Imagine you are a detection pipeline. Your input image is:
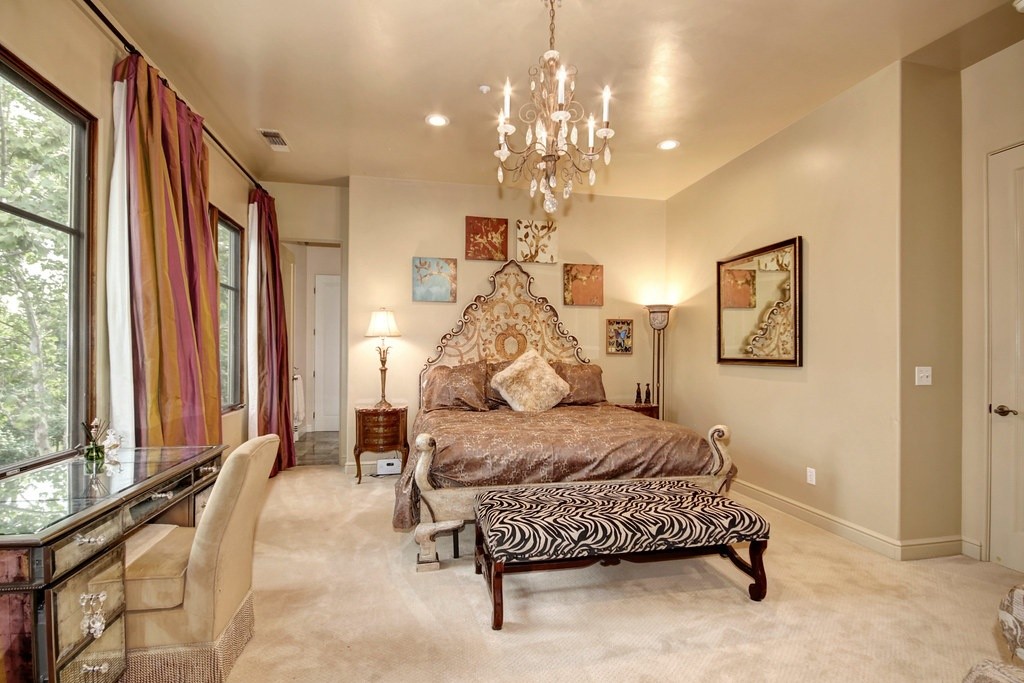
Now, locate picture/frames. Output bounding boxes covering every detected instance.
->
[604,317,635,357]
[712,234,805,370]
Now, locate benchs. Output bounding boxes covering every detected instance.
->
[465,478,782,633]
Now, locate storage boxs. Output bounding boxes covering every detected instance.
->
[376,457,404,477]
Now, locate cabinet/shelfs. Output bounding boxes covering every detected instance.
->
[0,443,233,683]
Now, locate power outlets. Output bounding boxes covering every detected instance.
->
[806,466,815,485]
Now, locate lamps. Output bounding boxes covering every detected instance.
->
[491,0,620,213]
[363,305,402,411]
[645,304,674,420]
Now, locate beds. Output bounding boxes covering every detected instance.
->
[391,259,741,590]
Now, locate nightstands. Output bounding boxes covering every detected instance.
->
[350,404,410,486]
[614,403,660,421]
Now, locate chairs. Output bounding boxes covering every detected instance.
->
[56,429,281,681]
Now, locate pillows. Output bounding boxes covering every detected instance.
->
[489,347,571,413]
[419,357,488,414]
[555,355,607,405]
[488,354,561,410]
